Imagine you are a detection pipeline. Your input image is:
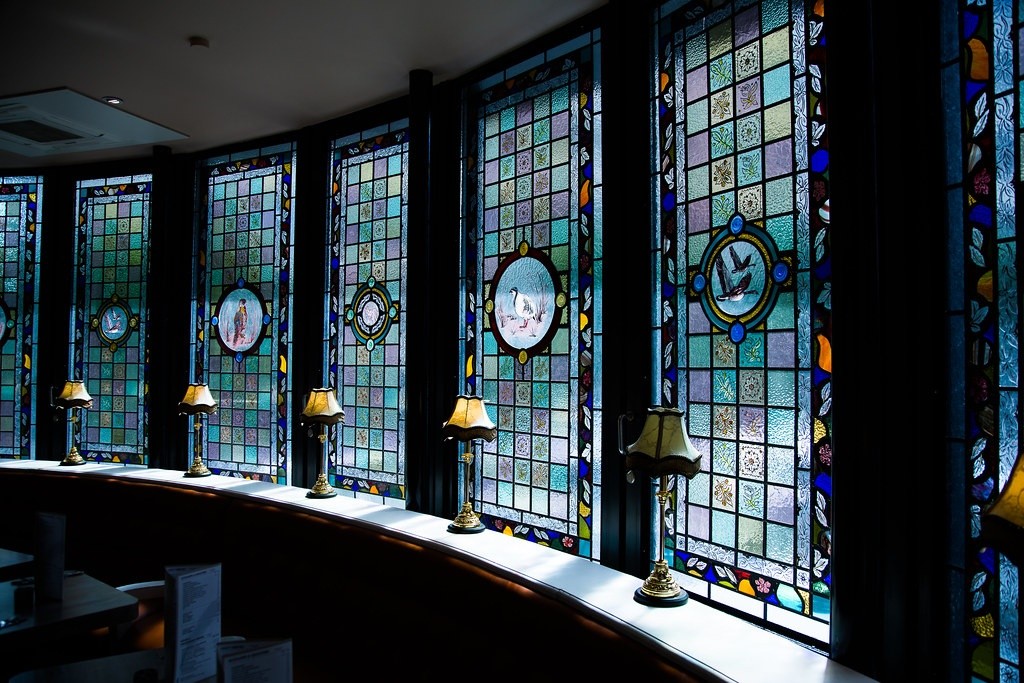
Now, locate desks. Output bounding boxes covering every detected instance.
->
[0,547,247,683]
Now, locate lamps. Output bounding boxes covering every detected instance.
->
[626,406,704,609]
[56,380,94,466]
[442,395,497,535]
[177,383,220,478]
[980,452,1024,683]
[299,388,346,499]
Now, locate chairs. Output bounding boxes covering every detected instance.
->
[92,579,166,654]
